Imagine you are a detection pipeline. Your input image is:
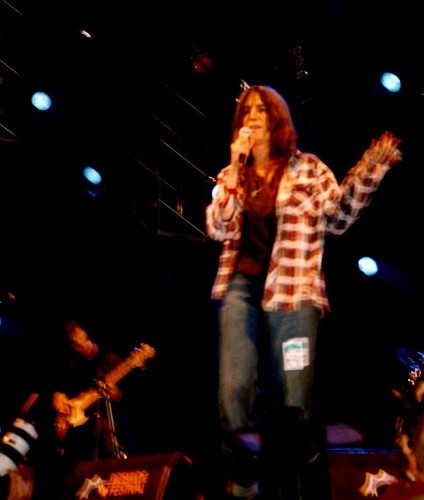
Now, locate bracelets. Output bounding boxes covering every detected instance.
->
[220,183,240,208]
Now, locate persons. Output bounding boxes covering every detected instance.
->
[206,83,400,432]
[52,326,126,459]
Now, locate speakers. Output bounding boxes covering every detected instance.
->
[73,453,194,500]
[330,448,409,500]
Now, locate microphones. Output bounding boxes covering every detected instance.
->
[238,127,251,167]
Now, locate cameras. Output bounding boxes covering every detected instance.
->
[1,394,55,480]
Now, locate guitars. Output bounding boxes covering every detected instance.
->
[54,341,155,440]
